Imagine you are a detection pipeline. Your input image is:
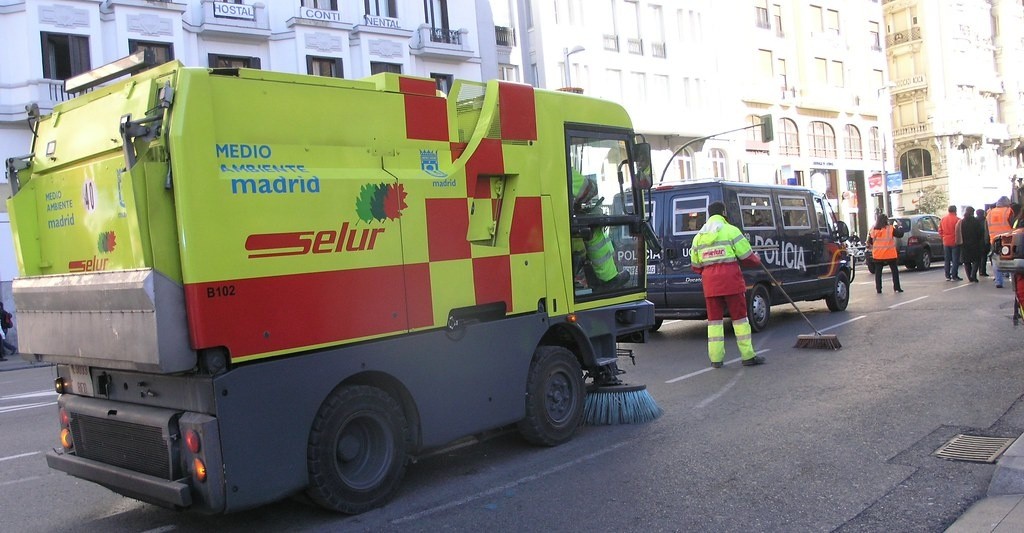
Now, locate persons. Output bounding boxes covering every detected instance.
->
[571,166,630,294]
[961,207,984,282]
[634,149,651,189]
[690,202,766,368]
[975,196,1021,288]
[850,232,861,247]
[0,301,17,361]
[868,214,904,293]
[938,205,963,281]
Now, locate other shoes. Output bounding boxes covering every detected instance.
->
[0,358,8,361]
[946,277,951,281]
[742,356,765,366]
[968,278,978,281]
[996,285,1002,288]
[980,273,989,276]
[606,269,630,289]
[877,289,881,293]
[951,277,963,282]
[894,288,903,292]
[11,347,16,354]
[710,361,723,368]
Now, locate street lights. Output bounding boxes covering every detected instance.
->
[564,46,585,86]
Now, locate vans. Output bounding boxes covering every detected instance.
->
[610,175,855,333]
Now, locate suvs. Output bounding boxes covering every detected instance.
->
[864,214,964,271]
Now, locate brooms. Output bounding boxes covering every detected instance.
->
[758,260,843,352]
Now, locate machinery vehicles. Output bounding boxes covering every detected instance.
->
[5,50,660,519]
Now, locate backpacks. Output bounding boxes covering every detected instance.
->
[4,312,14,328]
